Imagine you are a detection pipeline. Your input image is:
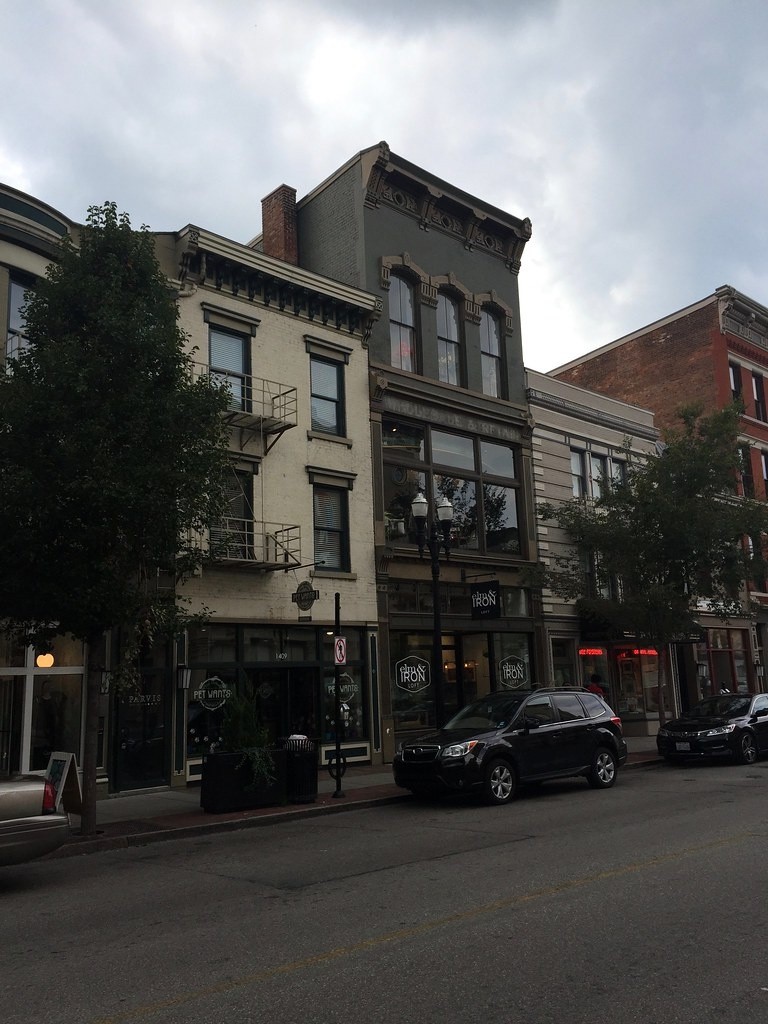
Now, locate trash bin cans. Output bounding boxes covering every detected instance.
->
[273,735,323,804]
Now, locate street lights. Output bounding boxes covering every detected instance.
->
[411,489,454,730]
[755,660,764,693]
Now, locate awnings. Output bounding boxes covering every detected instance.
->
[578,615,705,649]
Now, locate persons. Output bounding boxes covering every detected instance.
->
[588,674,604,701]
[719,682,730,695]
[120,684,146,752]
[38,681,59,755]
[460,667,477,707]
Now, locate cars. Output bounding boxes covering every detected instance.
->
[657,693,768,764]
[0,771,69,867]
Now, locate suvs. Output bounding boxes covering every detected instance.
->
[392,686,628,805]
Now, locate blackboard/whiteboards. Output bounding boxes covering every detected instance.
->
[45,752,71,807]
